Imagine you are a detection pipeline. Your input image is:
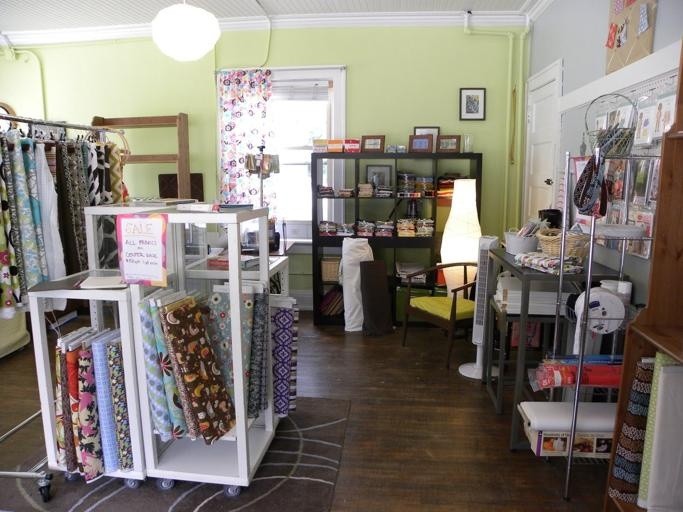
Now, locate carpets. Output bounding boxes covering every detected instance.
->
[0,397,351,512]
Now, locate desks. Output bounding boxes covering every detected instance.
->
[485,247,628,454]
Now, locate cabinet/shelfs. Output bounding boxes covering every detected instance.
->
[312,153,482,326]
[545,148,661,502]
[605,38,683,512]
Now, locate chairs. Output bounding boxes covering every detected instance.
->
[402,262,478,369]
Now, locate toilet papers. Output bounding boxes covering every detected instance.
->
[571,289,626,354]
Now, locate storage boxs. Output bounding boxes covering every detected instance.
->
[312,139,361,153]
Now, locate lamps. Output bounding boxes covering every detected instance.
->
[440,179,482,338]
[149,0,221,64]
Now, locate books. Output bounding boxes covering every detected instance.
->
[176,202,254,212]
[80,276,129,289]
[128,199,198,207]
[207,254,260,269]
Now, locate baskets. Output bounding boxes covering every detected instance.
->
[535,228,590,263]
[583,92,638,156]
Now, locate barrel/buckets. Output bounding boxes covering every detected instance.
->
[505,228,539,256]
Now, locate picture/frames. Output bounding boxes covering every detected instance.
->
[409,127,460,154]
[459,88,485,120]
[362,136,385,153]
[366,165,391,186]
